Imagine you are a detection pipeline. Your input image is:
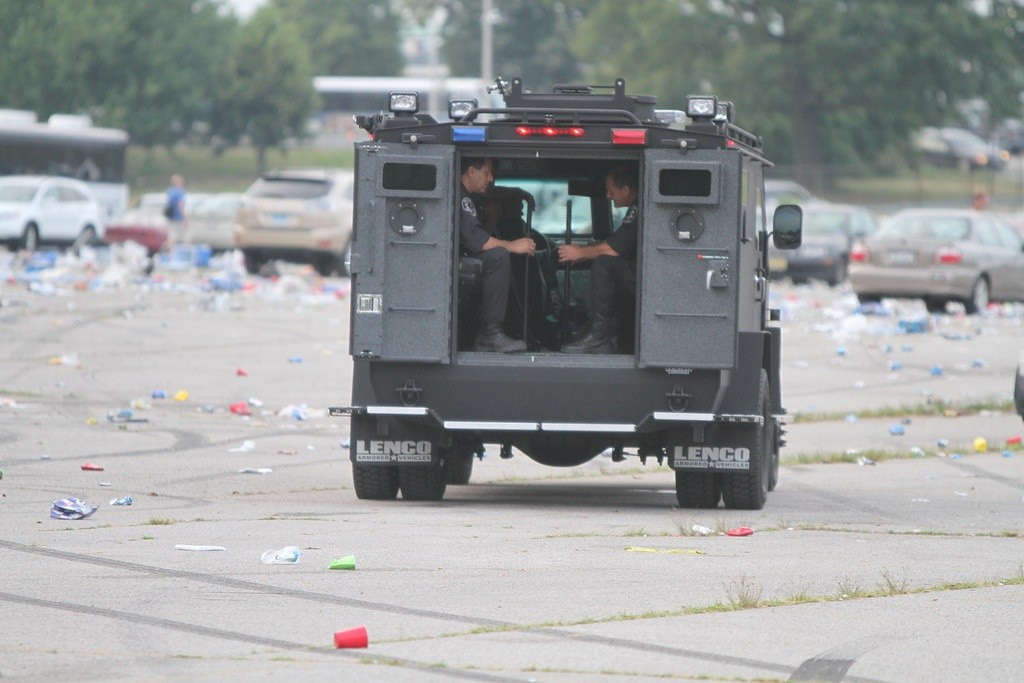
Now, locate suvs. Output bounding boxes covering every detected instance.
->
[234,168,354,276]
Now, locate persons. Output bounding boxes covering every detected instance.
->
[164,174,192,249]
[457,157,536,353]
[557,165,638,355]
[971,186,989,209]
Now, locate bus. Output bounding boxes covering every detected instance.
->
[0,110,131,249]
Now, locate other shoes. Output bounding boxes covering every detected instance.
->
[560,330,611,354]
[474,324,527,353]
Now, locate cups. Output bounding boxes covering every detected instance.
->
[329,555,355,570]
[973,437,987,452]
[334,627,367,650]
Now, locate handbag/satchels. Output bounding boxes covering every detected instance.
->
[164,205,174,218]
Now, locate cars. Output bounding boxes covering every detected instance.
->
[756,177,870,285]
[916,126,1013,169]
[851,207,1022,314]
[111,187,239,256]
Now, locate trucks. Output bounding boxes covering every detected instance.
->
[349,78,806,515]
